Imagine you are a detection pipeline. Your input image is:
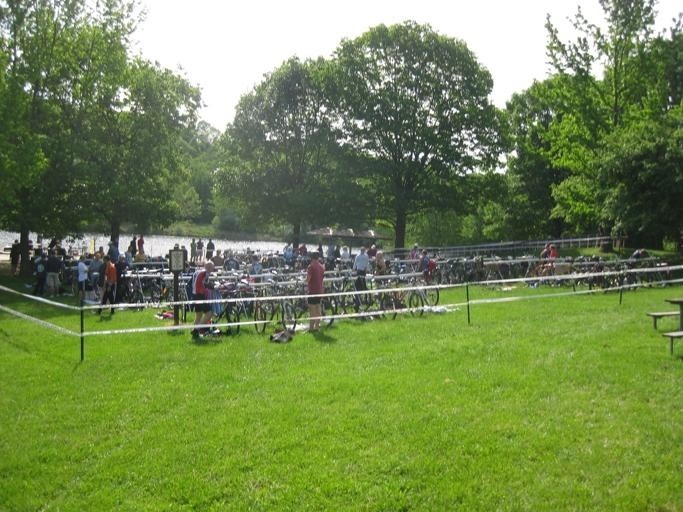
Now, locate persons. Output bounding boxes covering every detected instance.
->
[190,259,220,341]
[538,243,550,267]
[8,232,436,317]
[540,244,558,275]
[626,248,648,291]
[298,249,325,333]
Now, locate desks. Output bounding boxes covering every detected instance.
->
[664,299,683,331]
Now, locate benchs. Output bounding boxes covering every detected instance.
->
[662,331,683,356]
[646,311,680,330]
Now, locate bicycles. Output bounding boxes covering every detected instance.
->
[30,256,672,334]
